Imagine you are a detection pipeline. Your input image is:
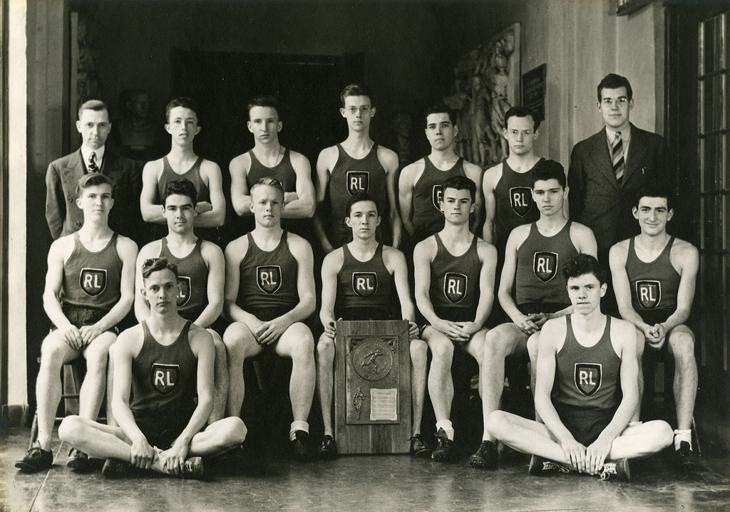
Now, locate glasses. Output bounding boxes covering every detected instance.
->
[602,97,628,108]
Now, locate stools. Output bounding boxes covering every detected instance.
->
[27,354,108,457]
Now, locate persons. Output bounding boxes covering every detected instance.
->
[608,184,698,478]
[316,84,403,254]
[399,103,483,251]
[134,177,229,467]
[486,254,674,479]
[223,177,316,463]
[568,73,674,319]
[45,100,141,416]
[413,176,506,469]
[57,258,247,478]
[483,106,566,325]
[105,90,168,181]
[14,174,138,472]
[316,193,433,460]
[140,97,226,253]
[455,39,511,169]
[469,160,597,471]
[229,95,317,255]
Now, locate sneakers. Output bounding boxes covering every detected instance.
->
[67,447,98,471]
[183,457,205,481]
[287,430,315,461]
[673,440,704,481]
[319,434,336,458]
[409,427,632,481]
[102,458,130,476]
[15,440,53,472]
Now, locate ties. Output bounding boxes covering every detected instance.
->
[610,130,627,186]
[87,152,100,174]
